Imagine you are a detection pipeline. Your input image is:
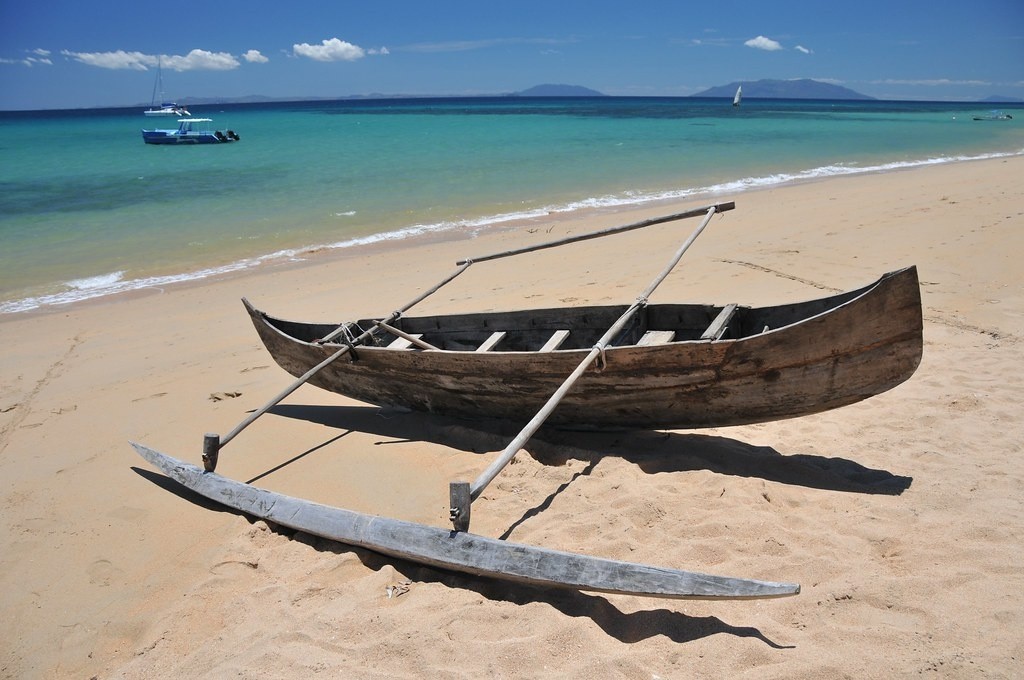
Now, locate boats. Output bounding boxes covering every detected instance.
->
[973,112,1013,121]
[141,118,240,145]
[240,263,922,435]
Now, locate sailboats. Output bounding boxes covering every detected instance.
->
[733,85,742,107]
[144,55,191,119]
